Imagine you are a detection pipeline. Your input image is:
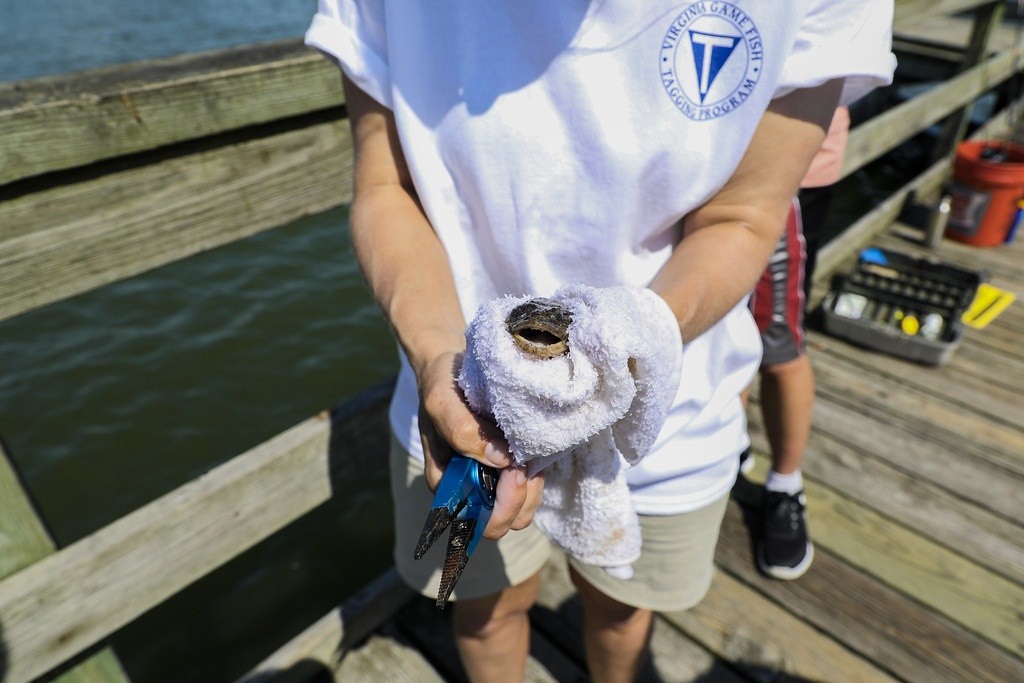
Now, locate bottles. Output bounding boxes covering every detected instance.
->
[925,196,951,248]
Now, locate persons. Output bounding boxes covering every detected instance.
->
[738,197,815,580]
[304,0,899,683]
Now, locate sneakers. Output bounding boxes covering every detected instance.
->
[739,447,754,474]
[756,486,813,580]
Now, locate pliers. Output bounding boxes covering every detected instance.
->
[413,451,498,609]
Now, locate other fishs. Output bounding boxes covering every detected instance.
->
[506,297,574,361]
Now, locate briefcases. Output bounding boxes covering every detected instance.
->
[820,244,981,367]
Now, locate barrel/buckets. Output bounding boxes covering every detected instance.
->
[944,141,1024,249]
